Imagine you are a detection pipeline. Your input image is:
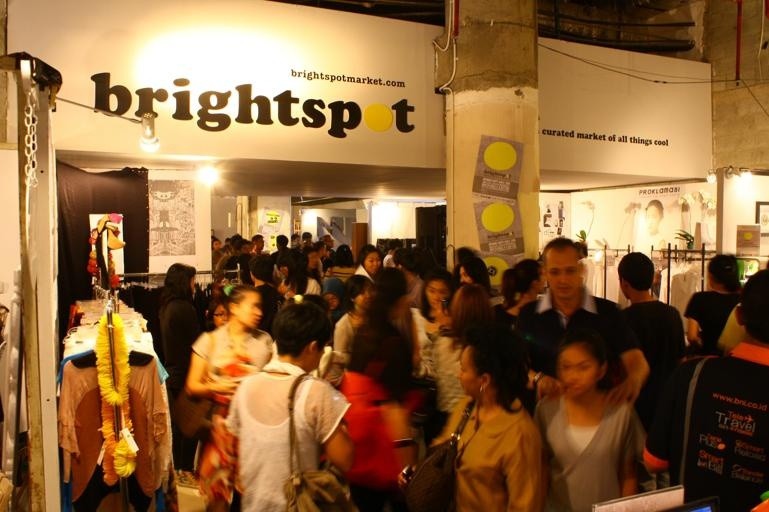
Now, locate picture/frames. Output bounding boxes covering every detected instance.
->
[756,201,769,236]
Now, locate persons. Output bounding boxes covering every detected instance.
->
[154,231,768,511]
[639,200,667,249]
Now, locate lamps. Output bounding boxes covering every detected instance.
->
[55,98,162,158]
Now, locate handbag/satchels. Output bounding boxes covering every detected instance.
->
[405,400,476,512]
[173,388,214,438]
[285,465,359,512]
